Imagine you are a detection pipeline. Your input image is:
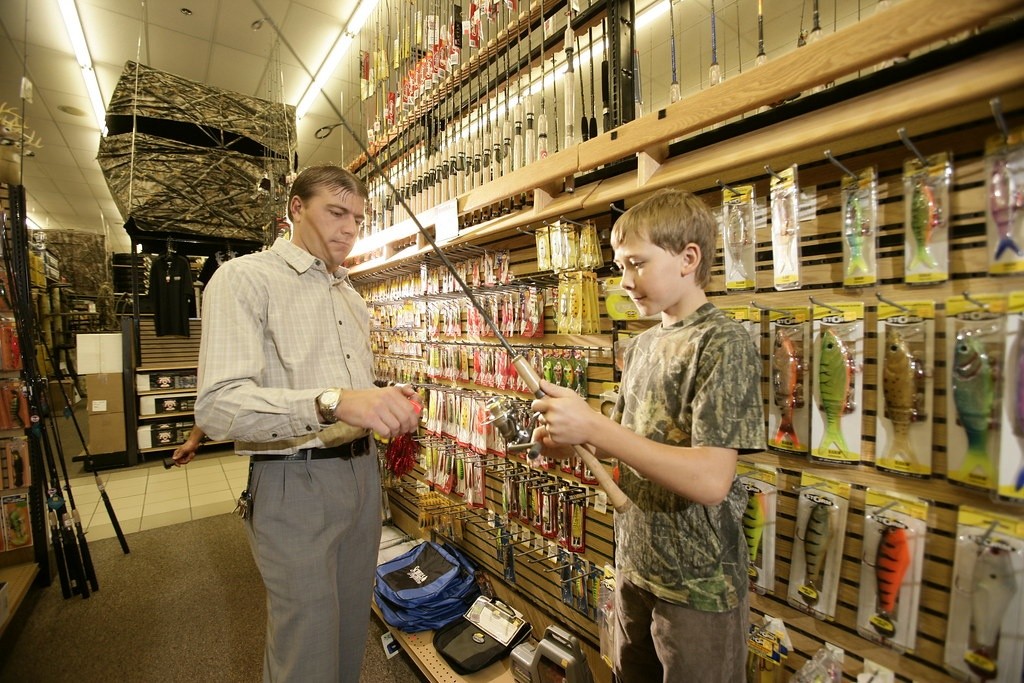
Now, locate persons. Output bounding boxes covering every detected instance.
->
[196,165,423,682]
[532,190,767,683]
[172,425,205,467]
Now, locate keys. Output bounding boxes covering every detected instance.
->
[230,490,252,521]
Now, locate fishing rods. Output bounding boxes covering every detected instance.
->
[0,180,131,598]
[254,0,634,518]
[337,2,910,271]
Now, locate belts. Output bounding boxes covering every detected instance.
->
[249,433,372,461]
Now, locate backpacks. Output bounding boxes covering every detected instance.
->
[373,540,479,634]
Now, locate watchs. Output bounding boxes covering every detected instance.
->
[319,387,344,423]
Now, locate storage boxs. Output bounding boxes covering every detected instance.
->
[77,333,101,375]
[35,256,45,274]
[36,272,47,288]
[29,268,37,288]
[88,412,127,455]
[85,373,124,416]
[101,333,123,374]
[28,250,36,270]
